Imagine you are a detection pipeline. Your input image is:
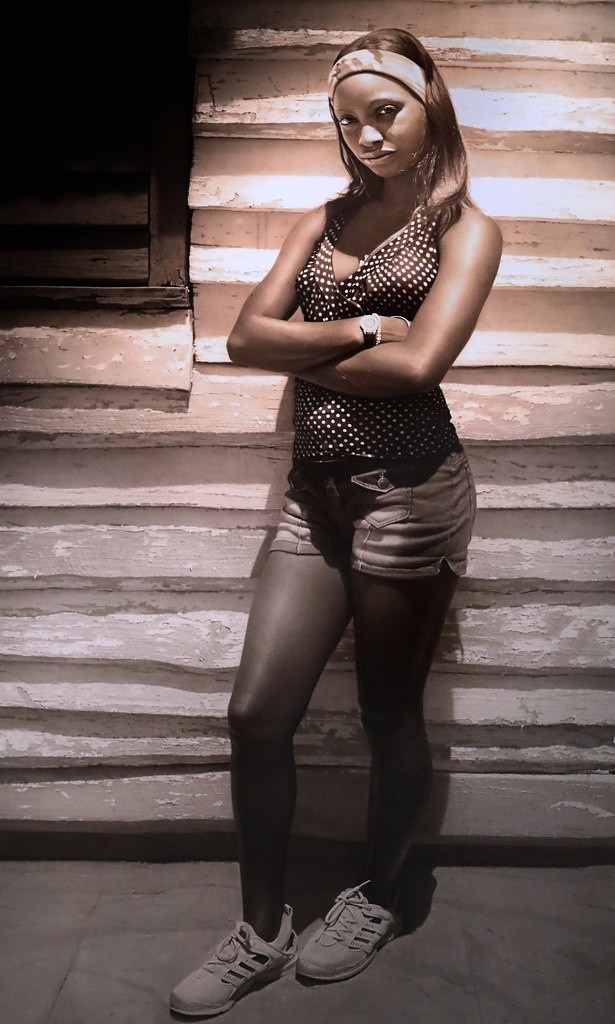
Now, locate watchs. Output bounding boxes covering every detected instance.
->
[358,314,378,348]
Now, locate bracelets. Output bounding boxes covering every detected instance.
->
[372,312,382,345]
[389,314,411,332]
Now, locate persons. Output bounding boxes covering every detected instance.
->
[166,26,502,1018]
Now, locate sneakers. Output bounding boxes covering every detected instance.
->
[166,901,299,1019]
[295,883,413,982]
